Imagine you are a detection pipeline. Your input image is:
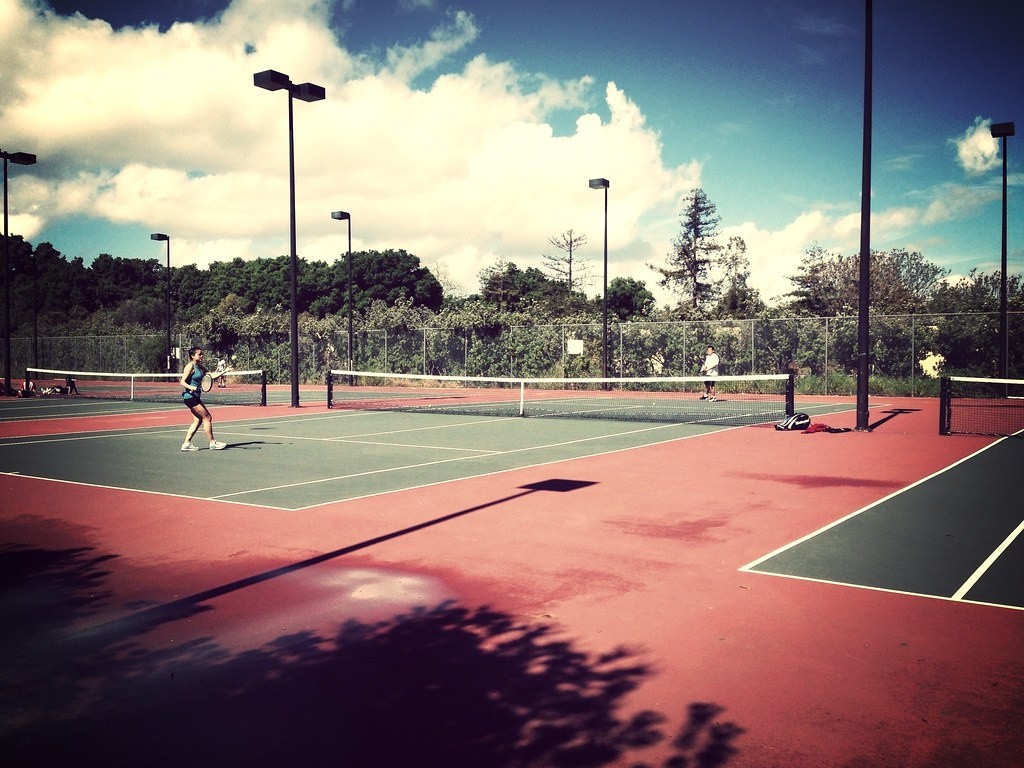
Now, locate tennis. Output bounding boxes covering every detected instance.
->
[232,355,237,360]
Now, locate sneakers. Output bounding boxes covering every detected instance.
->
[181,444,199,452]
[209,440,227,451]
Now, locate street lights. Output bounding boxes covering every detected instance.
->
[331,211,353,387]
[0,149,38,396]
[991,123,1015,400]
[589,179,610,389]
[254,70,326,409]
[151,233,171,382]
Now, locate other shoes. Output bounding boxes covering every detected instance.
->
[700,395,708,399]
[708,397,717,402]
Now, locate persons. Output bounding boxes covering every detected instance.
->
[214,356,226,387]
[180,346,233,452]
[17,375,80,398]
[699,346,719,402]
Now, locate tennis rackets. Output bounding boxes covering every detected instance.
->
[189,373,213,393]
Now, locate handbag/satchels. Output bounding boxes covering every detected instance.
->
[774,413,811,431]
